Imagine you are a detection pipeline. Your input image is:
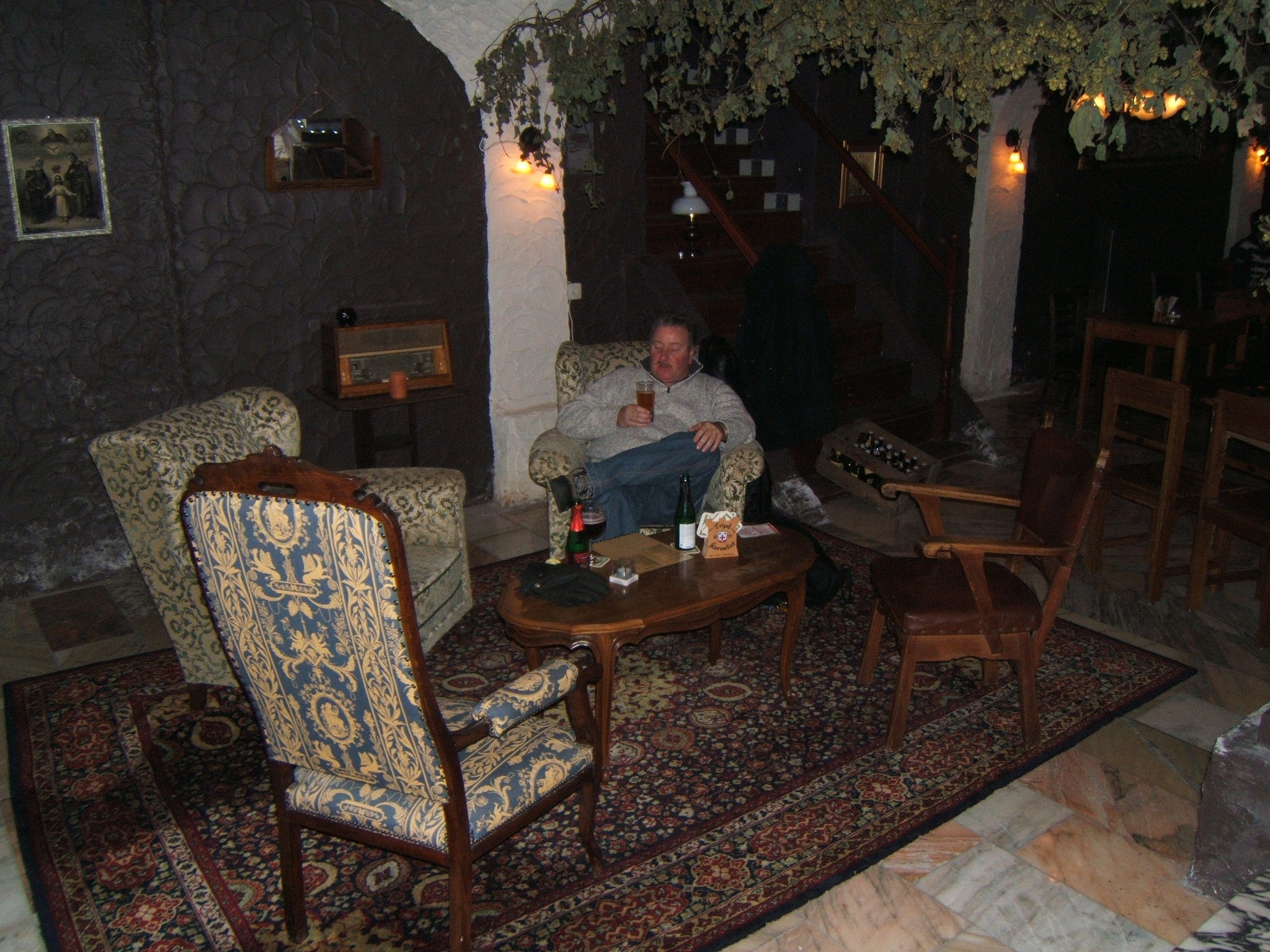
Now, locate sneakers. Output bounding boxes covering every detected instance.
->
[549,467,593,514]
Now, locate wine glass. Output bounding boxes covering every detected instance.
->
[577,497,606,563]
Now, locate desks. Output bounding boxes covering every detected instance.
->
[496,524,814,787]
[307,384,464,469]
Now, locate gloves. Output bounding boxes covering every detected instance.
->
[513,561,610,608]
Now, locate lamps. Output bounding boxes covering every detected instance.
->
[672,181,710,259]
[1072,87,1186,122]
[1004,128,1026,174]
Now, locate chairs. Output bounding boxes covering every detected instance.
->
[859,317,1270,752]
[178,444,605,952]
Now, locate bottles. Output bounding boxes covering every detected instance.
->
[673,474,697,551]
[830,431,926,488]
[567,498,590,568]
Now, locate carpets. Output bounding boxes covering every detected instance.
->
[0,509,1197,952]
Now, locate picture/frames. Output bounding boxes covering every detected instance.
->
[839,139,888,210]
[0,116,112,241]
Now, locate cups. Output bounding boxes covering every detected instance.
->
[388,372,407,399]
[1167,309,1180,326]
[636,379,656,423]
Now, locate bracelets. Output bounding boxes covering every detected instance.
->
[716,421,726,442]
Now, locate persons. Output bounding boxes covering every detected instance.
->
[548,312,757,683]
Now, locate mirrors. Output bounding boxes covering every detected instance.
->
[266,118,381,194]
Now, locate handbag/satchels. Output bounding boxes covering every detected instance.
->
[761,510,855,609]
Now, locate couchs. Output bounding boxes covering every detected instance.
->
[88,387,467,712]
[528,341,764,562]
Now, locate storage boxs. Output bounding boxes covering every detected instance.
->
[817,418,942,514]
